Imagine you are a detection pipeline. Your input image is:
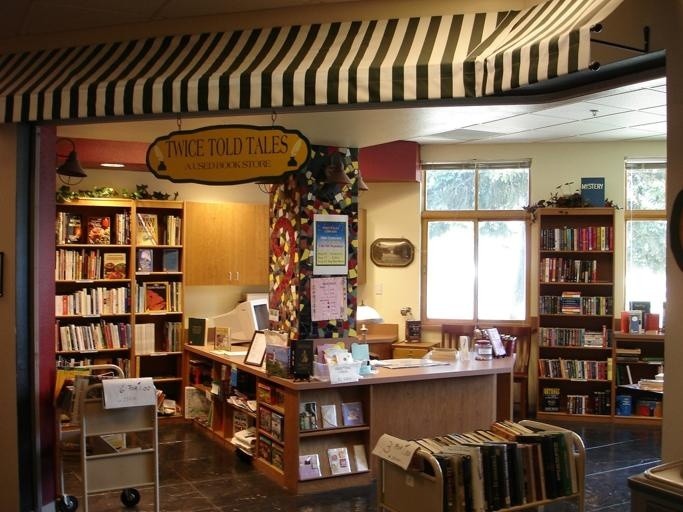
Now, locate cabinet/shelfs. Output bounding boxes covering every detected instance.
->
[374,419,586,512]
[56,196,186,418]
[391,339,439,361]
[180,338,373,499]
[532,204,663,427]
[183,200,270,286]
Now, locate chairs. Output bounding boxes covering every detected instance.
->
[439,322,531,419]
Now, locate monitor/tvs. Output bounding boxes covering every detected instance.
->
[212,298,270,345]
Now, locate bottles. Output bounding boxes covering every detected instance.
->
[474,339,493,361]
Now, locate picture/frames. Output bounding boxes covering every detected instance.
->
[243,330,266,367]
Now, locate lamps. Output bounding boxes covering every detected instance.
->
[55,137,87,186]
[323,151,351,186]
[355,299,384,340]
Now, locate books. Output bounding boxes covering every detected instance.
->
[263,343,290,379]
[298,453,321,481]
[370,418,578,512]
[213,325,230,351]
[187,317,207,345]
[342,402,363,427]
[539,225,664,416]
[290,337,313,374]
[298,401,317,430]
[183,358,285,471]
[328,447,352,474]
[319,404,338,430]
[54,210,182,464]
